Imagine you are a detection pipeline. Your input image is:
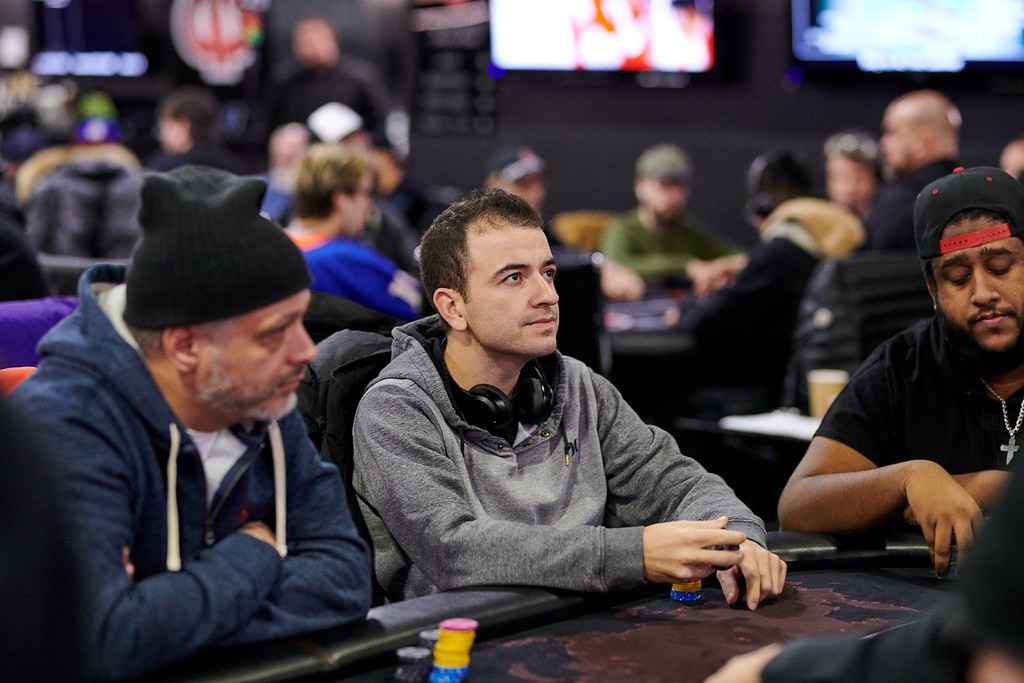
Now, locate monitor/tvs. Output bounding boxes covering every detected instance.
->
[490,0,730,90]
[789,0,1024,90]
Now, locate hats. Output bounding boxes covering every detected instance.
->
[913,166,1024,259]
[120,167,308,327]
[634,146,696,184]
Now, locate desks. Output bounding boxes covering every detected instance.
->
[607,284,693,358]
[140,526,960,683]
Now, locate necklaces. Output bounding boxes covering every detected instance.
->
[980,375,1024,464]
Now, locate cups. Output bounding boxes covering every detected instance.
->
[806,369,850,417]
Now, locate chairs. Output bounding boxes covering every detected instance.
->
[669,257,926,472]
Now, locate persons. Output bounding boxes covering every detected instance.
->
[482,147,646,303]
[282,142,423,314]
[777,164,1024,576]
[679,89,1024,427]
[597,138,755,293]
[0,167,377,665]
[353,184,788,609]
[700,435,1024,683]
[0,17,409,306]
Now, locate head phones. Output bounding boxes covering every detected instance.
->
[439,335,557,446]
